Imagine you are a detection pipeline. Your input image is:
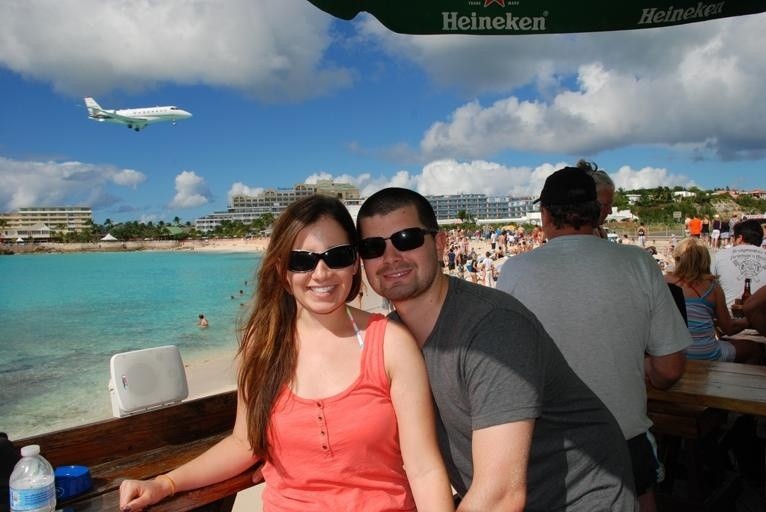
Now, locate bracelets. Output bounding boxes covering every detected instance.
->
[156,474,176,498]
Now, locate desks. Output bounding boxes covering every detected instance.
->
[646,361,766,416]
[0,388,264,511]
[720,332,766,361]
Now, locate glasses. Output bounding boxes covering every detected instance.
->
[358,228,438,259]
[288,243,356,273]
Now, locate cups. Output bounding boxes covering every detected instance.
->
[54,464,93,499]
[734,298,742,305]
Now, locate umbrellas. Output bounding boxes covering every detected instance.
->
[100,233,118,241]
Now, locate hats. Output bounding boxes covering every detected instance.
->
[533,167,598,206]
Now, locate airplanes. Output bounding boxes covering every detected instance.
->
[72,95,195,134]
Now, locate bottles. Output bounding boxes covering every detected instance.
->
[743,276,752,303]
[8,444,57,512]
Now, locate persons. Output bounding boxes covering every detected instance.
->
[732,284,766,339]
[711,220,766,320]
[445,224,547,286]
[662,236,762,363]
[120,195,455,512]
[578,159,616,238]
[360,188,640,511]
[684,213,749,250]
[497,167,692,511]
[198,313,208,329]
[606,221,674,270]
